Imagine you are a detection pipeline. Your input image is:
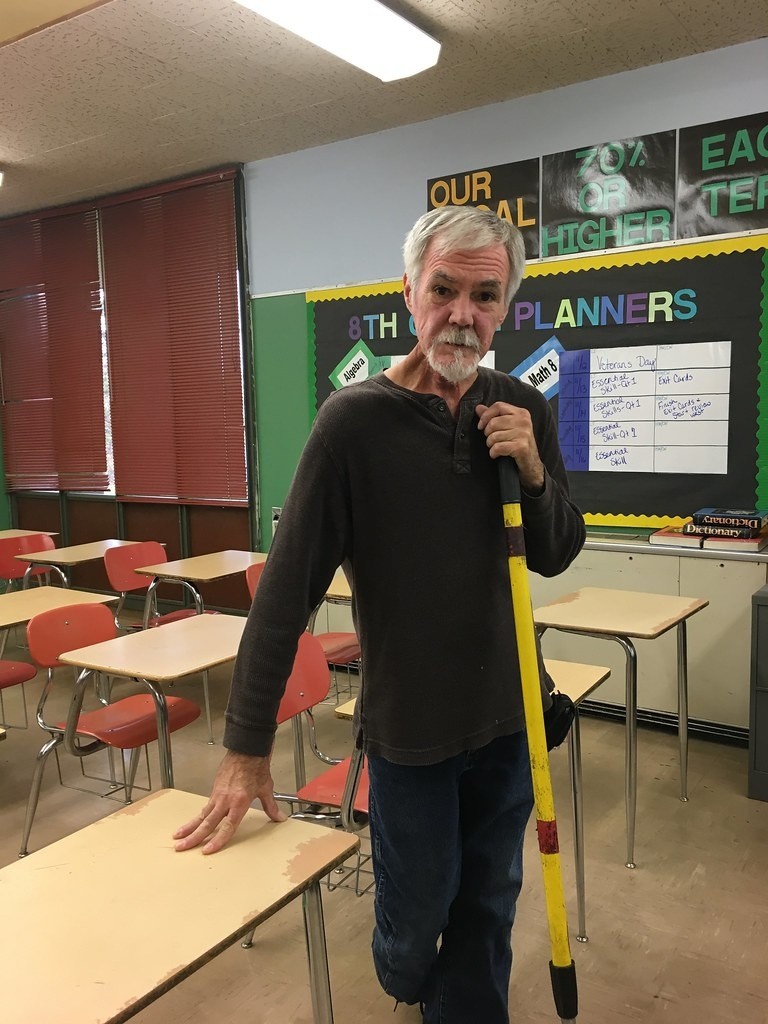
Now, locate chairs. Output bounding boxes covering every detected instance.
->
[19,602,202,857]
[106,541,221,688]
[0,535,55,595]
[246,562,362,704]
[238,628,369,948]
[0,658,37,728]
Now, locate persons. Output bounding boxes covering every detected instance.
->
[174,205,586,1024]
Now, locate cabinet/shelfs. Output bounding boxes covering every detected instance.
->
[748,584,768,802]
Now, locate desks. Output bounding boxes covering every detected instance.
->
[0,530,710,1024]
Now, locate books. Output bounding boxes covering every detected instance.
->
[648,508,768,552]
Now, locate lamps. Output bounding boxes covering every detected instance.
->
[232,0,442,82]
